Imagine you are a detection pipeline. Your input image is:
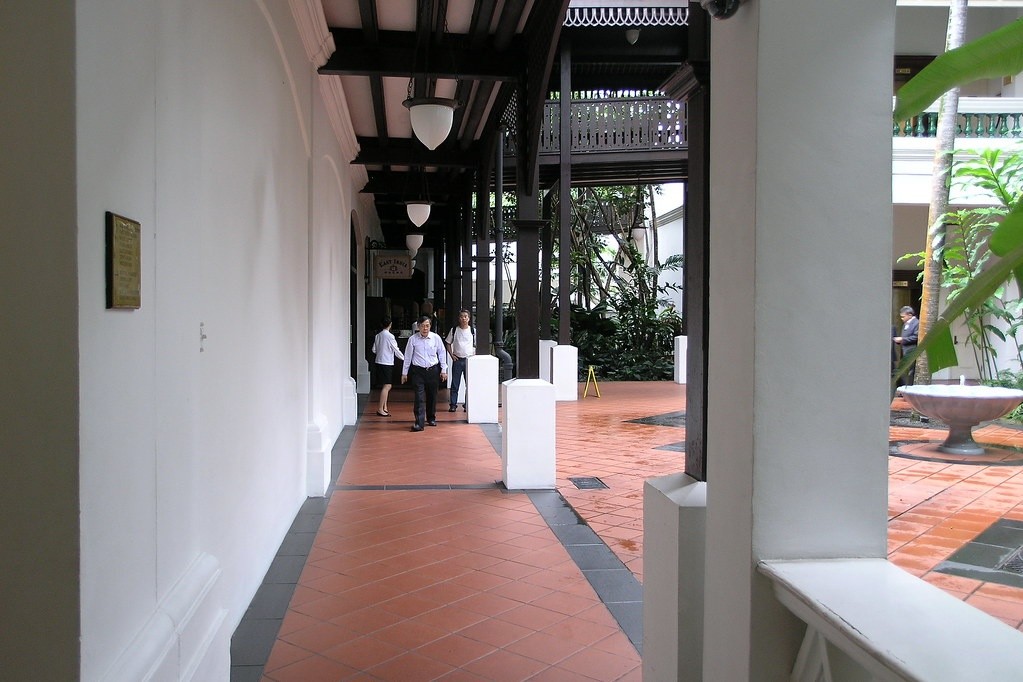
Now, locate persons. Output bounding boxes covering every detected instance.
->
[891,307,920,386]
[445,308,477,412]
[401,316,448,432]
[371,316,405,416]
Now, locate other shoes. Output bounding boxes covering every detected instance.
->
[448,404,457,411]
[383,410,389,413]
[463,407,467,412]
[376,410,392,417]
[413,424,423,431]
[429,421,435,426]
